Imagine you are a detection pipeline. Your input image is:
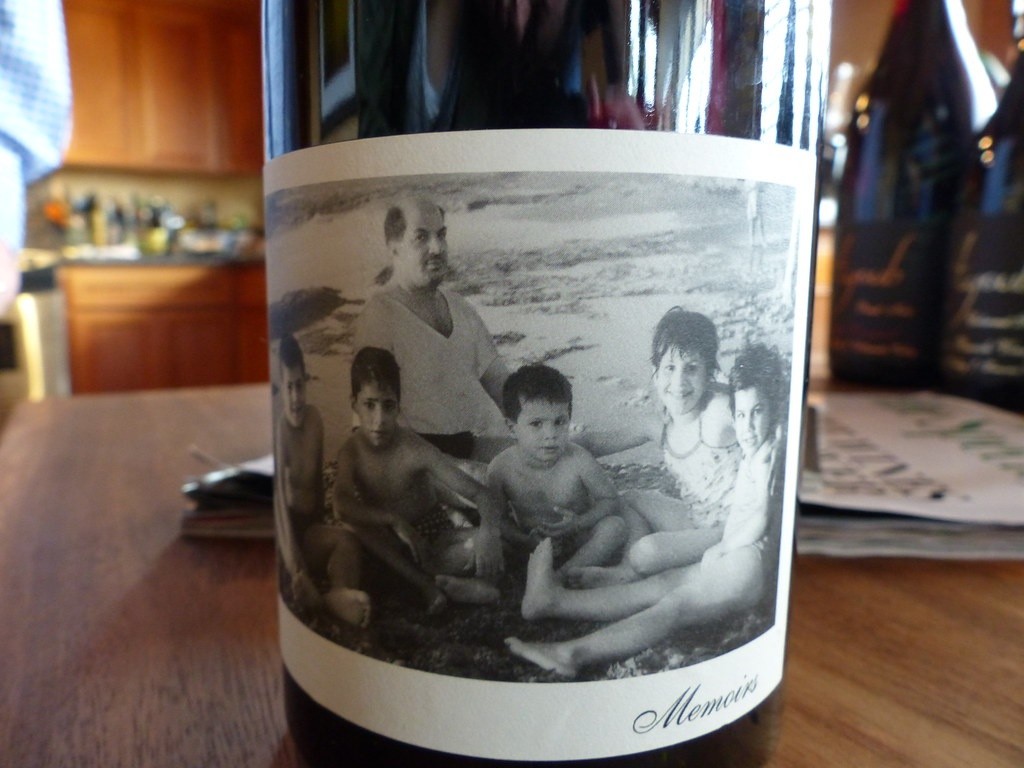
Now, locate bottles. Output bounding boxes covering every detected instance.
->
[932,10,1024,409]
[829,0,998,389]
[259,0,834,768]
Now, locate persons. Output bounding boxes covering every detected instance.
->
[353,197,512,509]
[270,309,790,678]
[0,0,74,312]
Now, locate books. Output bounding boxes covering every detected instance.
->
[795,390,1024,561]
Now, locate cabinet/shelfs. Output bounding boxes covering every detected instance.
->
[59,0,261,174]
[60,264,270,394]
